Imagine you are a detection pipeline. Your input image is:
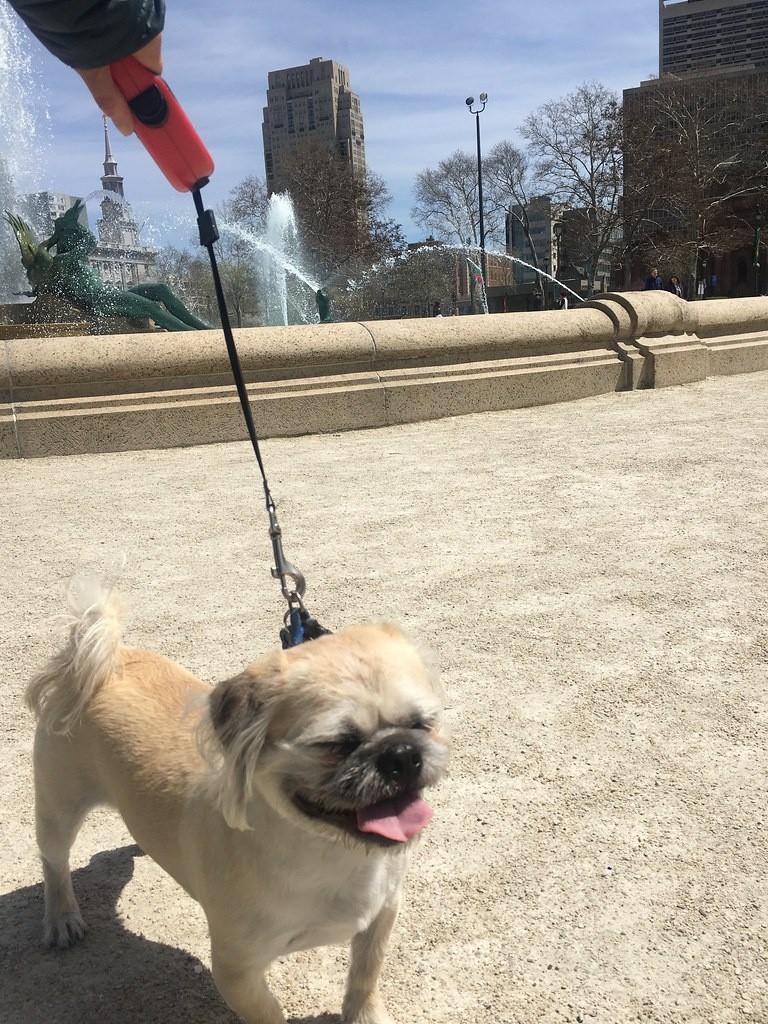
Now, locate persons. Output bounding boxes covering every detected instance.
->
[49,217,212,332]
[532,288,542,310]
[8,0,164,136]
[669,277,684,298]
[556,291,568,309]
[433,302,443,317]
[645,267,664,291]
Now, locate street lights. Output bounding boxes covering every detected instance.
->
[466,91,487,312]
[553,223,564,302]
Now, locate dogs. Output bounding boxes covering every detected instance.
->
[21,594,455,1024]
